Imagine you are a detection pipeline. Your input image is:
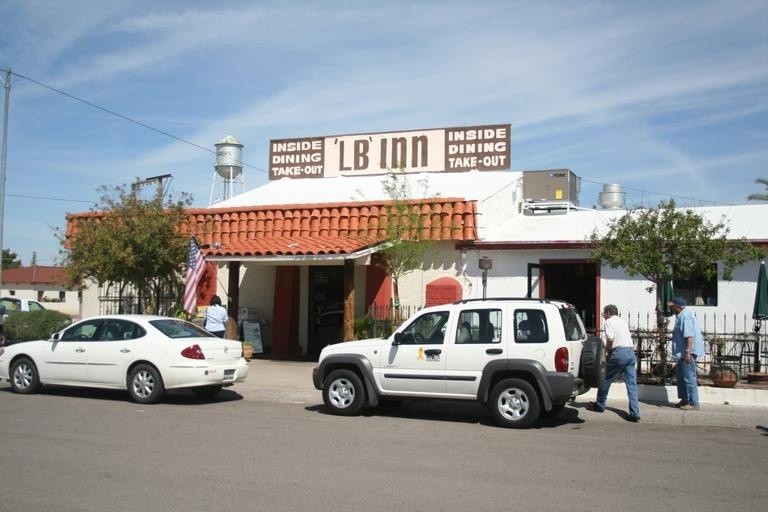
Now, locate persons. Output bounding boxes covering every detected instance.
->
[584,304,641,422]
[202,294,229,340]
[670,295,704,409]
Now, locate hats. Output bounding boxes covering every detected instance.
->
[667,298,685,306]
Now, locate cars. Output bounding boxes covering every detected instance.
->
[0,312,249,401]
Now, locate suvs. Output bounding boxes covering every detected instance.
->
[312,296,606,426]
[0,297,48,346]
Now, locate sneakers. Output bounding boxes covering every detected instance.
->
[587,402,604,412]
[676,400,695,409]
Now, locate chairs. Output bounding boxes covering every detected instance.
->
[518,319,530,339]
[456,323,471,343]
[486,320,494,341]
[713,333,751,381]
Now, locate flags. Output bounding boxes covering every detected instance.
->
[182,234,208,317]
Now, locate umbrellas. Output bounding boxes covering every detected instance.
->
[660,264,675,359]
[751,259,768,372]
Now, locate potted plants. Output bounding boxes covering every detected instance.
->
[241,340,254,362]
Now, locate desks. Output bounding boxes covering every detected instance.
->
[633,349,652,377]
